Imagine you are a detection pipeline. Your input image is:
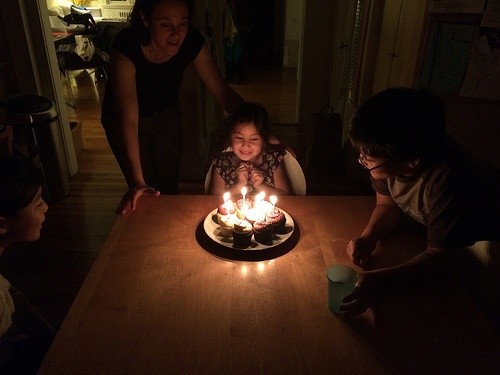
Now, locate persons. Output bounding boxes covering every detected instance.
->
[213,102,292,197]
[0,154,77,375]
[100,0,296,215]
[338,87,500,321]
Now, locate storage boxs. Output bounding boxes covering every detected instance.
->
[69,120,83,156]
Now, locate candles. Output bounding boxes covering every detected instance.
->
[223,187,277,224]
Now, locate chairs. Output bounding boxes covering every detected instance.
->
[205,145,306,194]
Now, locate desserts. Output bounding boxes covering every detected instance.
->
[218,199,286,246]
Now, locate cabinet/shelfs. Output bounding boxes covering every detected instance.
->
[373,1,427,93]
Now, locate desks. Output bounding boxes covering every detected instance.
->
[35,194,500,375]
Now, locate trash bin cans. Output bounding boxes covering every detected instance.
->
[70,122,84,155]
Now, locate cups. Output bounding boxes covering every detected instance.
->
[326,266,358,315]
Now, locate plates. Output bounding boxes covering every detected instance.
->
[203,203,294,250]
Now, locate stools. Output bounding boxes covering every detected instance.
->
[0,95,59,202]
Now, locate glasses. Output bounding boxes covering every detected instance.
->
[357,153,393,174]
